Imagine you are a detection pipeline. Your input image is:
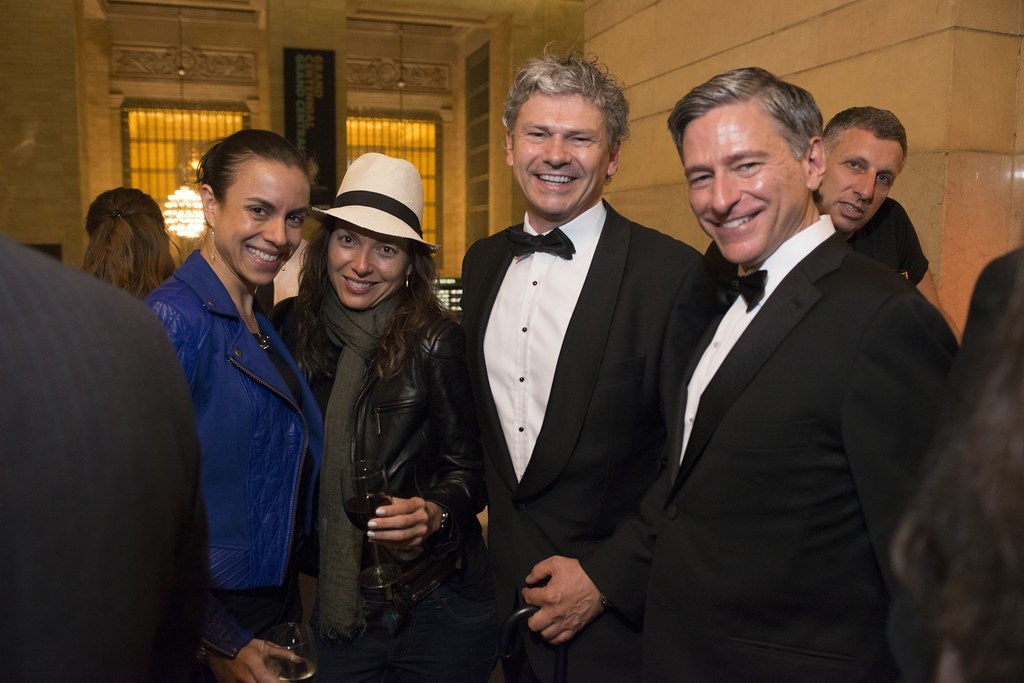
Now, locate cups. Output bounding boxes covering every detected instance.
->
[263,622,317,683]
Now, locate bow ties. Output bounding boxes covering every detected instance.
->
[504,227,576,260]
[714,264,767,313]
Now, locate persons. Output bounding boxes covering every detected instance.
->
[459,39,715,683]
[267,151,498,683]
[641,67,1024,683]
[83,187,173,299]
[0,233,201,683]
[148,130,324,683]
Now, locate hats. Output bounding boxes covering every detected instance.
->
[310,151,443,254]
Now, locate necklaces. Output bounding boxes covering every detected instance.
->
[239,308,269,349]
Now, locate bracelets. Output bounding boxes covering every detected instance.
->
[600,591,611,609]
[442,507,449,527]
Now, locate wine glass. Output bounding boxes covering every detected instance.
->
[339,460,401,589]
